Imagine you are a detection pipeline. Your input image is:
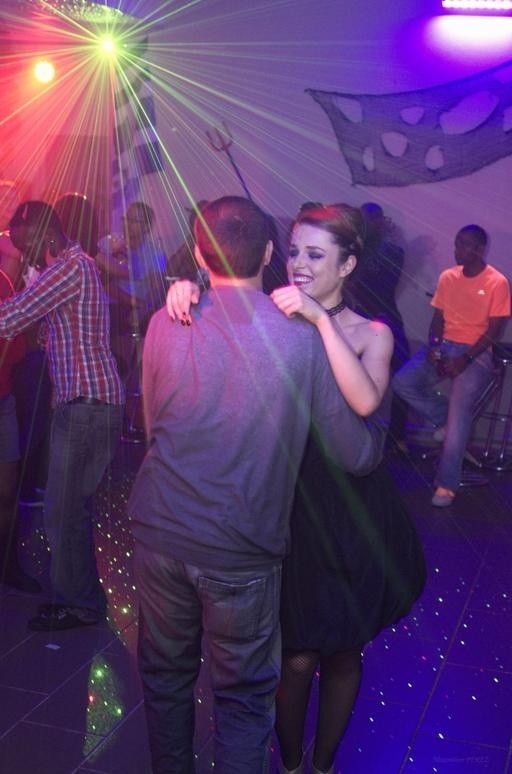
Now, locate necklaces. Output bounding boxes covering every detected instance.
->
[325,300,346,316]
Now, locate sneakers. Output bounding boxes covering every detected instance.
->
[29,608,88,629]
[431,487,454,506]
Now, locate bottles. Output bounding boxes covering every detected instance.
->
[434,350,447,377]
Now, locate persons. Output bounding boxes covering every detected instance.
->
[389,224,510,508]
[100,201,166,368]
[3,200,127,630]
[2,192,135,589]
[343,202,412,458]
[163,201,426,772]
[126,193,394,773]
[166,200,207,284]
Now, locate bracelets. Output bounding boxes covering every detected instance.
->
[464,354,472,364]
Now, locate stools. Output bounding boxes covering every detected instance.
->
[420,341,512,471]
